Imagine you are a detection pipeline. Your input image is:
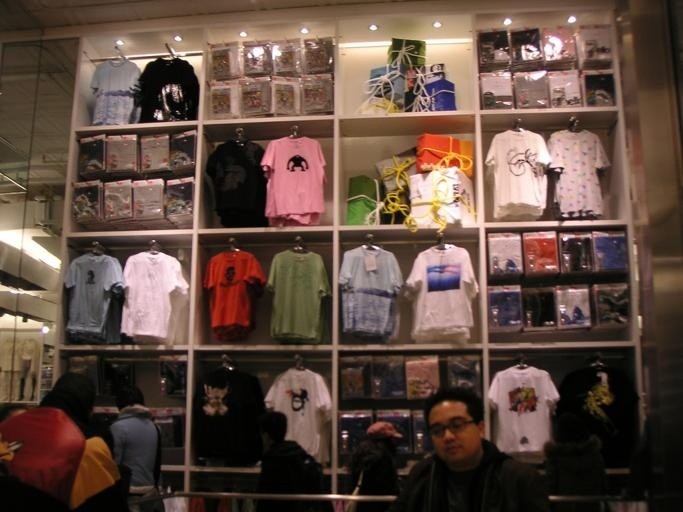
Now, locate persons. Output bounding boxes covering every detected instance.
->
[105,381,165,512]
[344,417,403,511]
[0,403,29,424]
[0,370,131,511]
[250,410,333,511]
[390,387,551,508]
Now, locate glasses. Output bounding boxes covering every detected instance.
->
[427,417,476,438]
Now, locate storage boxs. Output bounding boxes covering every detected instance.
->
[361,38,455,111]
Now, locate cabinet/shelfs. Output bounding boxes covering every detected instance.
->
[57,0,643,496]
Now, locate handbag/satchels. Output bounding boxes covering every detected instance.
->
[342,488,359,512]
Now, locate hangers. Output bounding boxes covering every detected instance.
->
[514,352,605,369]
[233,126,302,143]
[511,117,582,133]
[110,45,176,63]
[362,229,453,252]
[90,237,158,256]
[216,354,305,372]
[223,233,304,255]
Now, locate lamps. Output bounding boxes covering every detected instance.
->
[41,320,53,333]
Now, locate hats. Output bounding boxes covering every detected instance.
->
[366,420,403,439]
